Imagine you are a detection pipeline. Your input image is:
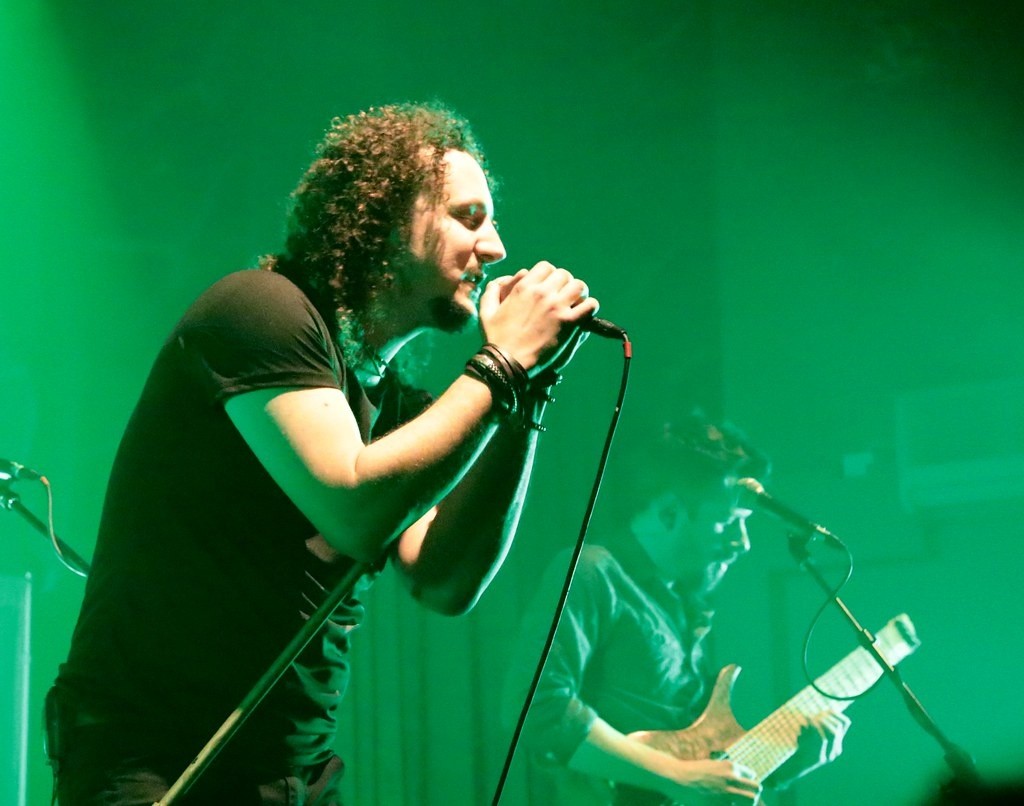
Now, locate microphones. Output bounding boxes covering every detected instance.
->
[0,457,42,481]
[578,314,627,339]
[731,478,845,548]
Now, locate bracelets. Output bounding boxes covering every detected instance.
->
[464,342,563,432]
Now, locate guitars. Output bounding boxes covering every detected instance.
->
[612,610,922,806]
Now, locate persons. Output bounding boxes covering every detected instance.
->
[41,102,600,806]
[503,415,852,806]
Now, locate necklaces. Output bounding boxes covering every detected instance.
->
[367,348,390,377]
